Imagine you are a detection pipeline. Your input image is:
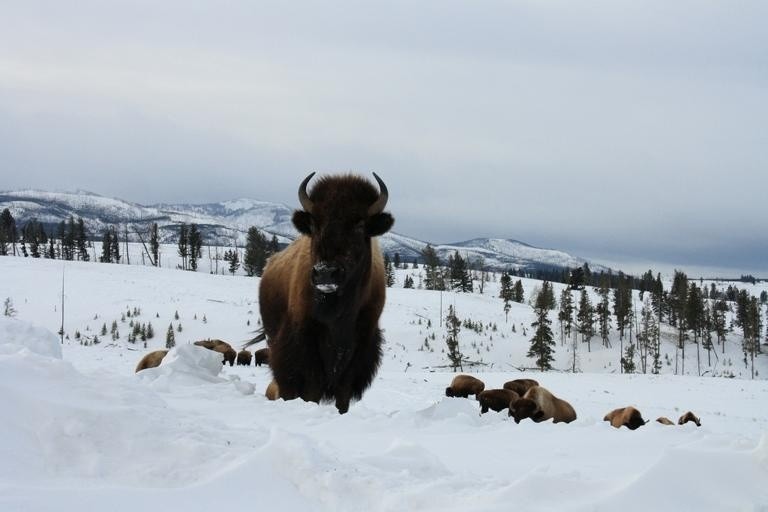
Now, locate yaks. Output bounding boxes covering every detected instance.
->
[678,412,701,426]
[242,172,394,413]
[446,375,485,400]
[135,350,168,372]
[237,348,252,366]
[194,338,237,366]
[255,348,270,366]
[603,407,649,430]
[477,388,519,415]
[503,379,576,424]
